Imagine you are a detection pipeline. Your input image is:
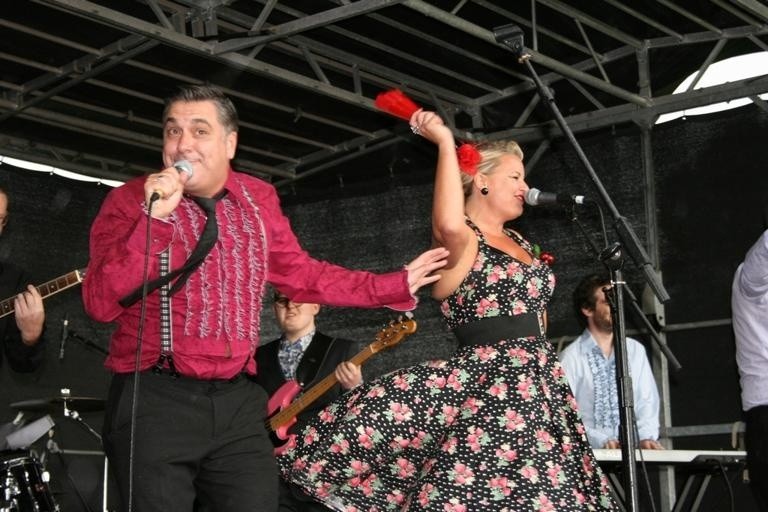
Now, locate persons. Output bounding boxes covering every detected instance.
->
[560,274,662,450]
[730,219,768,509]
[252,287,363,438]
[275,109,623,512]
[83,88,450,512]
[2,190,54,367]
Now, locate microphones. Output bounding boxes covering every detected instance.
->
[59,311,70,359]
[524,187,592,206]
[150,159,193,201]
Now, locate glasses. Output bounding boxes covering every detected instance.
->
[273,297,304,307]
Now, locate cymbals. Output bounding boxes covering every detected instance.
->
[11,395,107,415]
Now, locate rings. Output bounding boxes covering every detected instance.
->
[409,126,419,137]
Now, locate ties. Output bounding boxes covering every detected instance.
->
[118,189,227,308]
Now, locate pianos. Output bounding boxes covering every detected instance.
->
[594,448,745,467]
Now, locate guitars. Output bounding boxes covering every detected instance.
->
[266,311,416,453]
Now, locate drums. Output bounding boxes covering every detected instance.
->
[0,458,59,507]
